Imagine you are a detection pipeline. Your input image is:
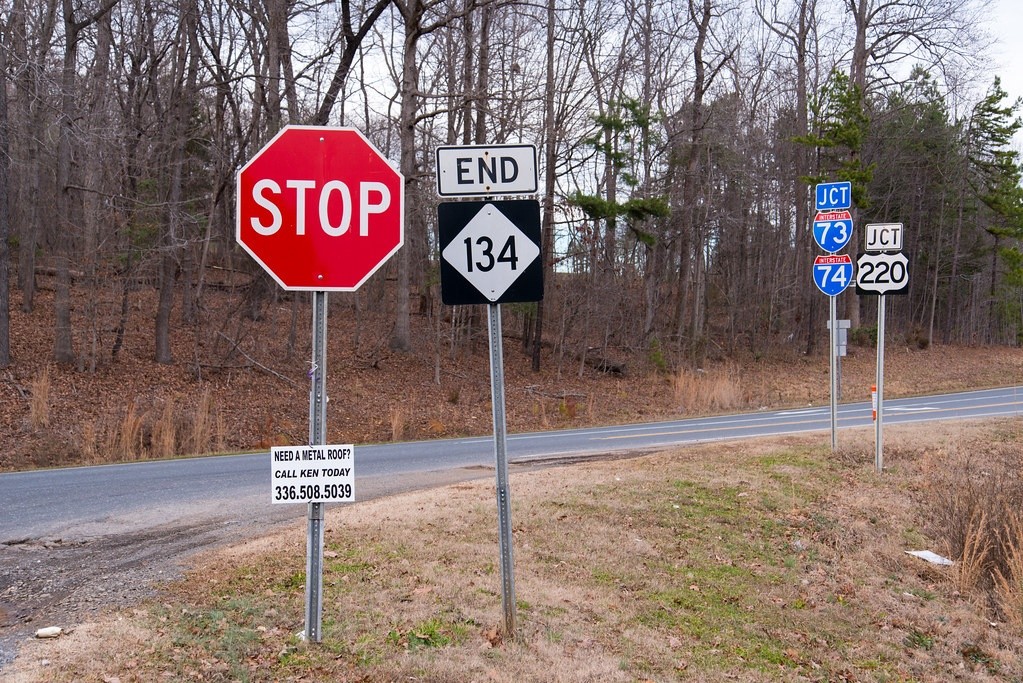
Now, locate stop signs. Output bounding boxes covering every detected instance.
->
[237,125,407,293]
